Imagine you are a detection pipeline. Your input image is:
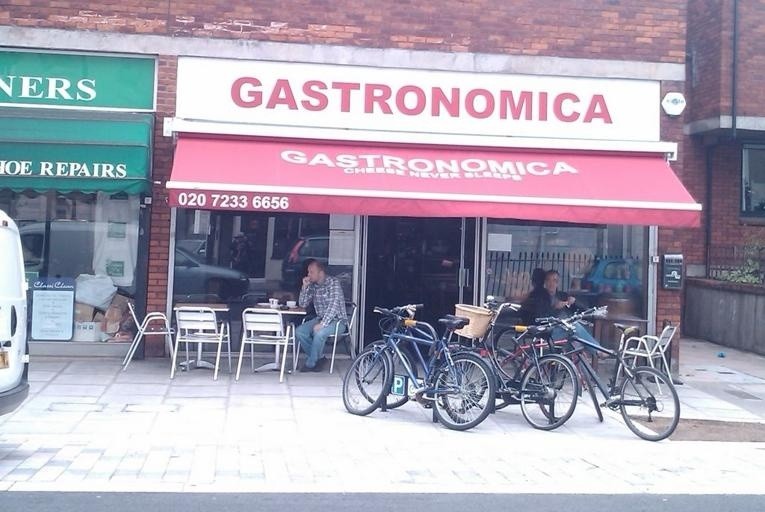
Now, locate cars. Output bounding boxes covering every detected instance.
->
[175,245,250,303]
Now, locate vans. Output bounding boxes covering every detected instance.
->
[0,209,31,418]
[16,220,130,287]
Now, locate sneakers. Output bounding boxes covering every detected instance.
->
[596,346,616,360]
[313,356,329,372]
[299,364,313,372]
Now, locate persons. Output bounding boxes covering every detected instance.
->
[228,231,252,278]
[525,269,617,361]
[295,259,348,373]
[241,216,266,274]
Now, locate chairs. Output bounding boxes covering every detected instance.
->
[121,298,359,384]
[530,308,681,397]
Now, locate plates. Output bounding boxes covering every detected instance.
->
[288,306,298,309]
[257,302,270,307]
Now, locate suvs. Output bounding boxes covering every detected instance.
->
[282,234,355,300]
[577,256,642,294]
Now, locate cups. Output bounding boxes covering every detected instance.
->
[286,301,296,306]
[269,299,278,308]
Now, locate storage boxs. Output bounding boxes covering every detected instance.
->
[72,294,135,343]
